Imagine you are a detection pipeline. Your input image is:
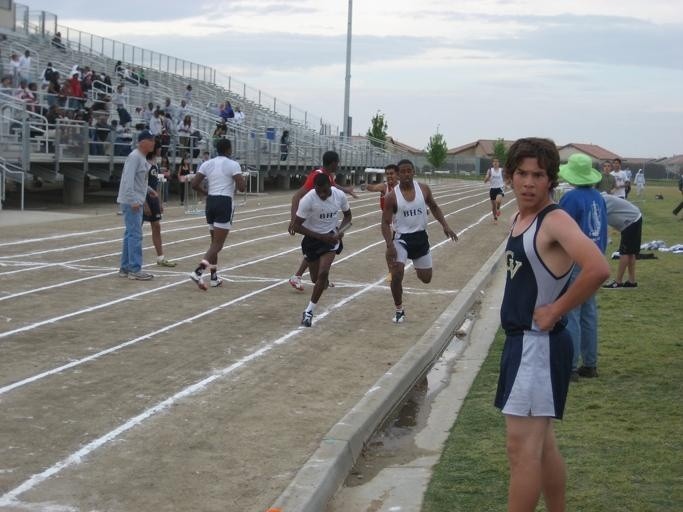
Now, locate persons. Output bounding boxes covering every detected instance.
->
[1,32,292,206]
[484,159,506,222]
[494,138,610,512]
[597,158,683,289]
[116,130,245,292]
[288,150,458,327]
[560,153,608,383]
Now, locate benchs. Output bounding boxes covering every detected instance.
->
[0,19,392,167]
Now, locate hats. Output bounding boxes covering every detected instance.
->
[557,153,602,185]
[138,130,155,141]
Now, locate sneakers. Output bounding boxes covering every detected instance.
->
[289,273,305,291]
[120,270,153,280]
[624,279,638,288]
[210,277,224,287]
[601,281,624,290]
[191,272,208,290]
[393,309,406,323]
[570,366,600,382]
[301,309,313,327]
[494,210,500,224]
[157,258,176,267]
